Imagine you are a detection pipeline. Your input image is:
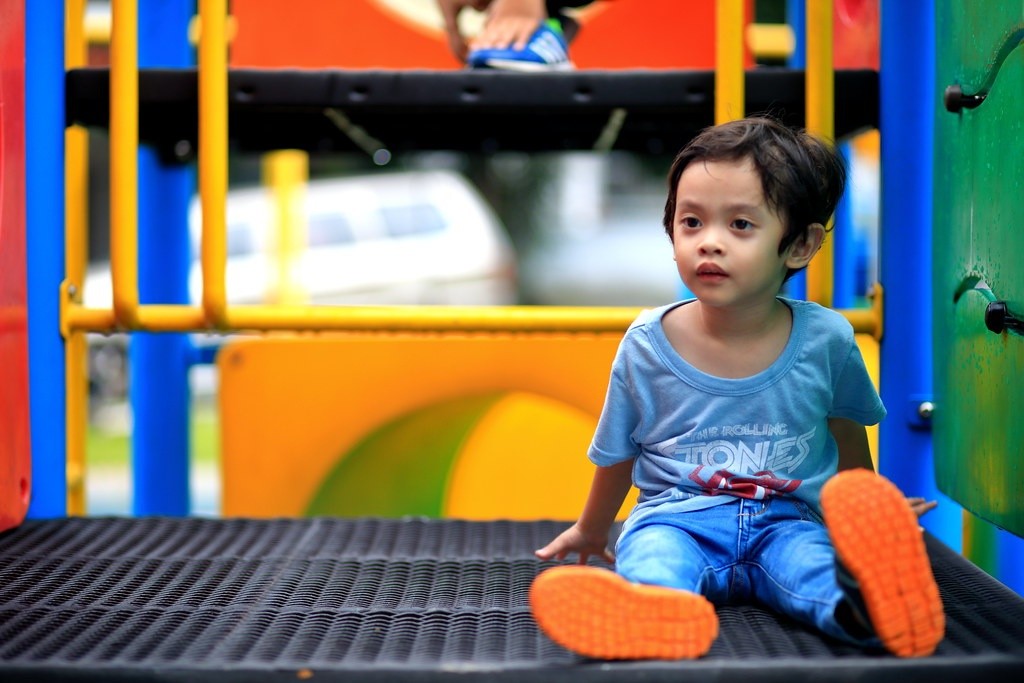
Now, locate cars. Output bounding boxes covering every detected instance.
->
[79,165,516,408]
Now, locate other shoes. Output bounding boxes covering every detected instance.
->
[820,469,946,658]
[530,565,719,660]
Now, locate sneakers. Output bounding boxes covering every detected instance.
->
[469,18,575,72]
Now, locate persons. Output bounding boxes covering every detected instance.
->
[439,0,600,74]
[529,116,947,659]
